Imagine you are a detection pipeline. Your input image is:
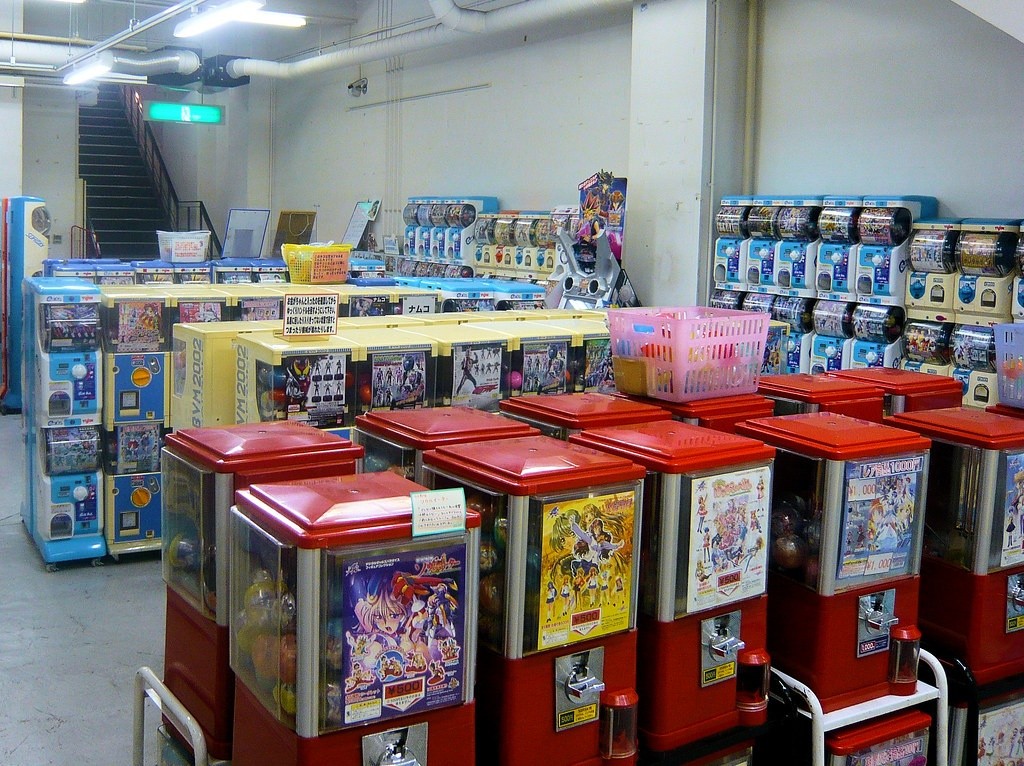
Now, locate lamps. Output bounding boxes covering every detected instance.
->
[0,0,112,85]
[172,0,307,38]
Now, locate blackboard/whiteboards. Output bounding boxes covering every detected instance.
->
[341,201,377,249]
